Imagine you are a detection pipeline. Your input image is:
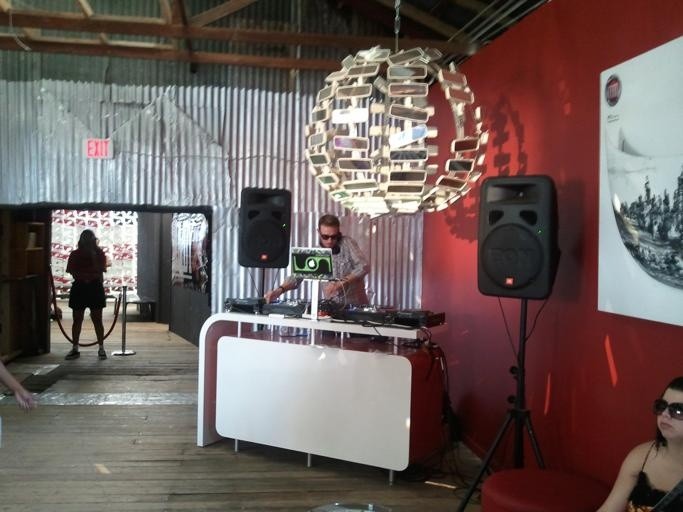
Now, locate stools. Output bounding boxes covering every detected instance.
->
[480,469,609,512]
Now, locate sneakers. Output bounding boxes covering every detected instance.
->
[98,350,107,359]
[66,349,80,360]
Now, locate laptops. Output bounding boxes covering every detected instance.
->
[290,247,348,283]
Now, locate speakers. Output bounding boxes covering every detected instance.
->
[476,174,560,299]
[237,188,292,268]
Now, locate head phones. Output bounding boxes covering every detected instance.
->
[319,232,342,254]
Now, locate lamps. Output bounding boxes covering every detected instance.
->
[304,0,489,216]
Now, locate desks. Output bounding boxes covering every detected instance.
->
[197,313,448,485]
[113,293,155,320]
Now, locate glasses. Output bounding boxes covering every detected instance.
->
[322,234,338,240]
[652,398,683,420]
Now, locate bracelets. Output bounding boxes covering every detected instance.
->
[278,285,286,293]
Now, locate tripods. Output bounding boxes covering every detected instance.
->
[456,299,545,511]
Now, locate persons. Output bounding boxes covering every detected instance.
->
[64,228,107,360]
[264,214,371,306]
[594,376,683,512]
[0,359,35,409]
